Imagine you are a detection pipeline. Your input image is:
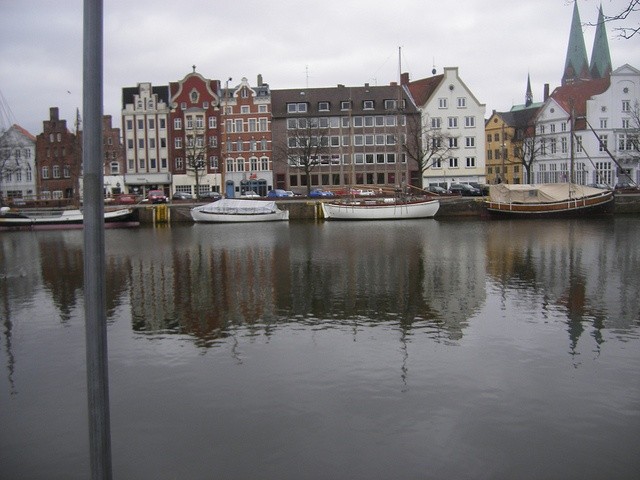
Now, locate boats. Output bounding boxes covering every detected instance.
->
[321,182,440,221]
[0,206,141,231]
[189,199,290,223]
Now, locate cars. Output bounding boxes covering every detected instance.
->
[267,189,295,198]
[172,191,192,200]
[586,184,614,190]
[307,189,334,198]
[423,186,453,196]
[200,192,222,199]
[614,183,640,194]
[243,191,260,198]
[111,195,135,205]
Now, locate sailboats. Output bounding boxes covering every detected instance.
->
[484,106,640,218]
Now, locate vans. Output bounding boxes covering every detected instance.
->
[356,188,375,196]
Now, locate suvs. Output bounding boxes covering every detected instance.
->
[449,183,481,196]
[147,190,167,205]
